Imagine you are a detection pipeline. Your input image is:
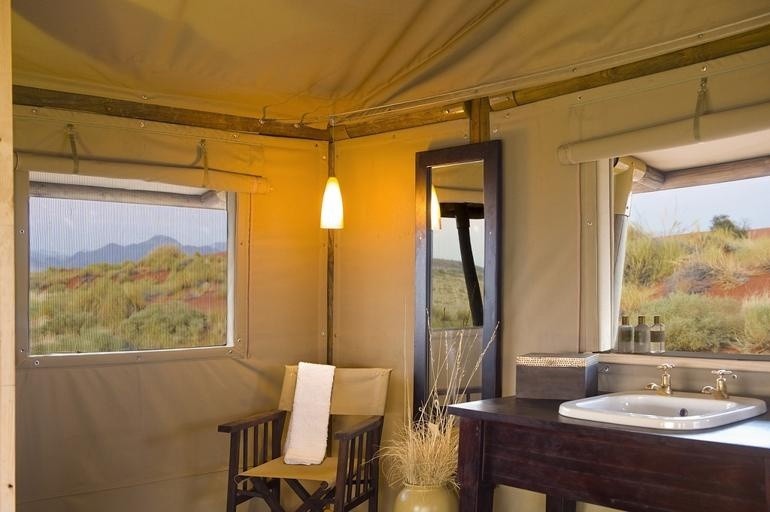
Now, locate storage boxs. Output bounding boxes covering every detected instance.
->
[514,350,599,401]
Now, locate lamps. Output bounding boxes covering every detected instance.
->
[320,133,345,231]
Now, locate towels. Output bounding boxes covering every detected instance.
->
[283,361,335,465]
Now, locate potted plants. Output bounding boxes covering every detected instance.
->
[387,317,499,511]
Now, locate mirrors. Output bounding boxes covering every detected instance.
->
[414,140,500,437]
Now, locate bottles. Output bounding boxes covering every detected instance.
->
[617,316,633,354]
[634,315,651,355]
[650,315,666,355]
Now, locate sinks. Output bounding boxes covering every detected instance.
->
[558,389,767,431]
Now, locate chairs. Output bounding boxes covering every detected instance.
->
[215,363,394,511]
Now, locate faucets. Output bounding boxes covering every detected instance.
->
[644,364,675,396]
[701,369,733,400]
